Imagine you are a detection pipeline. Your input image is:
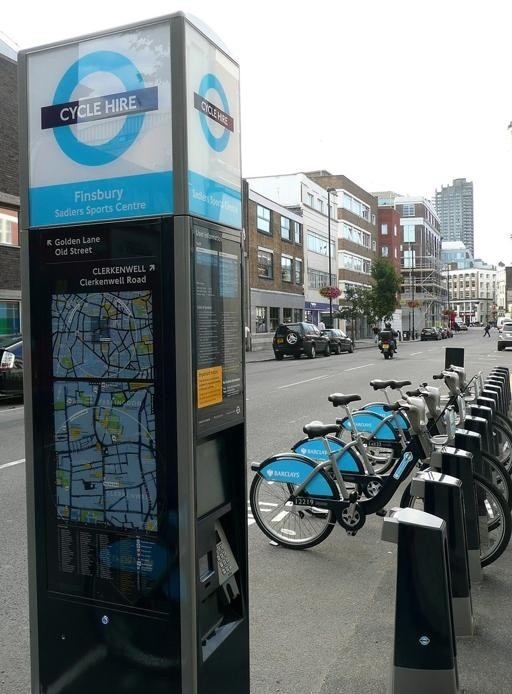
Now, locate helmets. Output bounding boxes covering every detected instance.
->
[385,321,392,327]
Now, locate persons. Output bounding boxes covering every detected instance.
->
[377,321,398,353]
[482,322,490,337]
[318,319,325,331]
[372,325,380,342]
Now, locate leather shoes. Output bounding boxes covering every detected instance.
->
[393,347,398,353]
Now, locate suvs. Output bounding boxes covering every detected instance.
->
[272,322,331,359]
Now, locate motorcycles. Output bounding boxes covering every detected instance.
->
[378,330,399,359]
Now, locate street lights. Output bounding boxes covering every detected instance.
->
[327,187,337,329]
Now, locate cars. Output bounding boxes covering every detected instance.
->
[497,317,512,351]
[457,321,467,330]
[0,335,23,400]
[320,328,354,354]
[421,327,453,340]
